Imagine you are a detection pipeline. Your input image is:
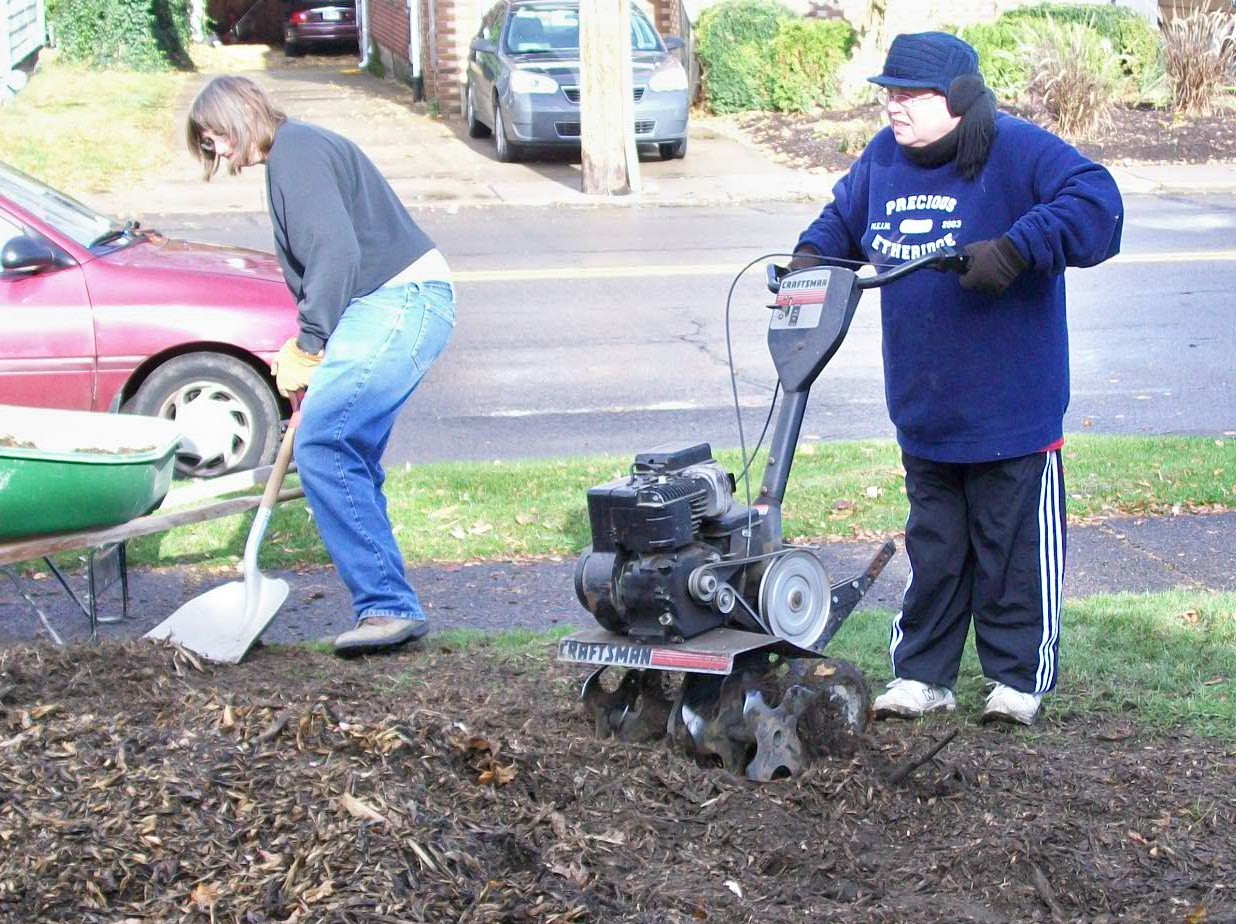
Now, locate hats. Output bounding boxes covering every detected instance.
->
[867,32,978,97]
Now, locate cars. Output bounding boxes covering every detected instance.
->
[465,0,690,164]
[0,160,307,482]
[278,0,360,56]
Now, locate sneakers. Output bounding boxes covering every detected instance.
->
[874,677,952,719]
[335,614,429,655]
[982,679,1043,726]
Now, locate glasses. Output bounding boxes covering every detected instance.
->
[876,87,936,108]
[199,126,232,153]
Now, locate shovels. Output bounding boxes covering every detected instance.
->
[141,413,303,664]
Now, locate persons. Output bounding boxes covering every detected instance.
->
[186,74,454,654]
[781,32,1124,725]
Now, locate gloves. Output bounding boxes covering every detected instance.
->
[959,236,1028,299]
[786,245,823,273]
[271,337,325,399]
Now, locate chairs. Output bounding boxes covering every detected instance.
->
[513,17,544,49]
